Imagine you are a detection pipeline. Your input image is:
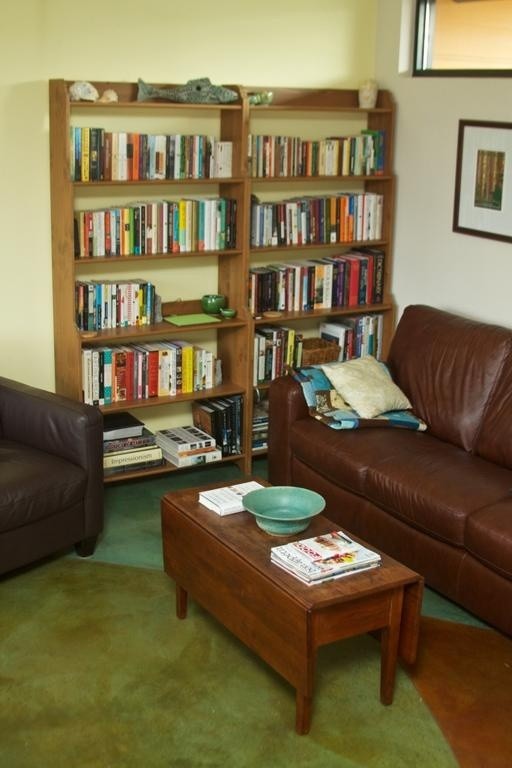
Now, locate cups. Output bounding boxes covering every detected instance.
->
[358,78,379,109]
[222,309,235,318]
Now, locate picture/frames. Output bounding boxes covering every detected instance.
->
[451,118,512,243]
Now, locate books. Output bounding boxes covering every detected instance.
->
[71,127,233,181]
[253,314,385,387]
[74,280,163,331]
[251,191,384,248]
[253,404,269,450]
[192,395,242,456]
[198,480,266,517]
[155,426,222,468]
[102,412,164,475]
[74,198,237,258]
[270,530,382,587]
[81,340,222,407]
[250,247,384,313]
[247,130,384,178]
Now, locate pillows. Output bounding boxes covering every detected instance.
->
[323,356,413,417]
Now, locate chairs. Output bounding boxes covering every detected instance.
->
[0,375,104,580]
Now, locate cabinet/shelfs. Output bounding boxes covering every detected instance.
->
[237,79,394,476]
[50,78,248,477]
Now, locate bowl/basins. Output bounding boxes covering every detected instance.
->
[202,294,226,313]
[243,485,327,536]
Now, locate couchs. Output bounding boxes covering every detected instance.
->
[267,304,512,643]
